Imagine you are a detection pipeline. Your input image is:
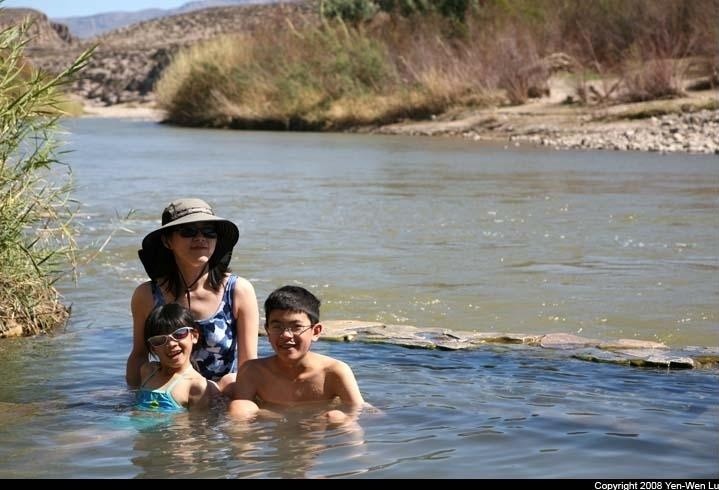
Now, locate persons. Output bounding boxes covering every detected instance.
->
[228,286,364,434]
[126,198,387,415]
[74,303,210,453]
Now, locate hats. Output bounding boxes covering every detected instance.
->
[138,198,239,279]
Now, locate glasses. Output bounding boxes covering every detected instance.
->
[176,225,220,239]
[266,324,313,335]
[148,326,194,348]
